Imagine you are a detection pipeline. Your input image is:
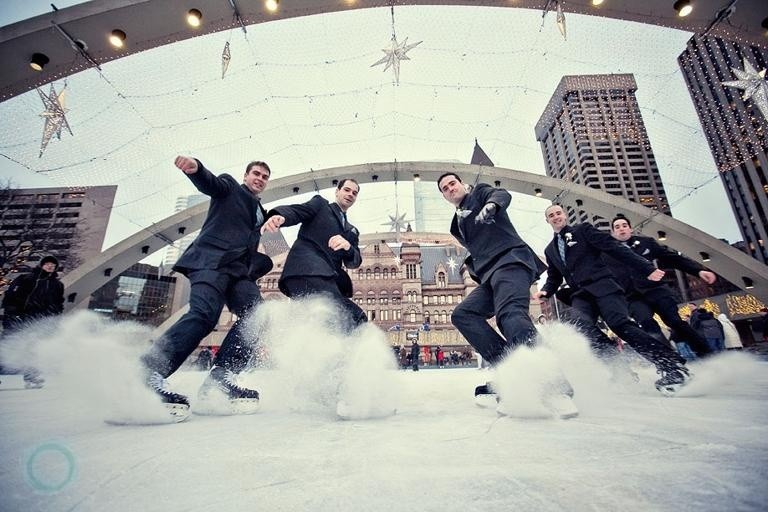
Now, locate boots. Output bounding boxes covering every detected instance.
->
[655,357,693,385]
[197,352,259,400]
[140,363,190,408]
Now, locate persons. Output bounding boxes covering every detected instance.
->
[0,255,65,385]
[437,172,574,407]
[689,303,699,331]
[136,155,259,408]
[399,348,407,369]
[435,346,469,368]
[410,338,420,371]
[696,308,725,354]
[685,315,690,324]
[533,204,693,396]
[717,313,743,352]
[258,178,368,336]
[611,216,716,362]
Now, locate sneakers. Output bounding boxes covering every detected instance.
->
[538,378,573,396]
[475,384,496,396]
[24,371,45,383]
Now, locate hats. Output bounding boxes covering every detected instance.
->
[41,256,59,270]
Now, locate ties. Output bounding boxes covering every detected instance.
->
[455,208,465,241]
[340,211,345,227]
[557,233,566,266]
[620,241,631,249]
[255,202,264,227]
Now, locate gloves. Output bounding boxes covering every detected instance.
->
[475,202,496,225]
